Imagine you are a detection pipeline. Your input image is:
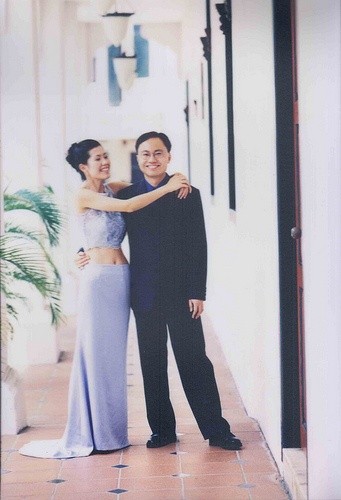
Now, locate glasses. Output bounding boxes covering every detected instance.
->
[138,149,168,161]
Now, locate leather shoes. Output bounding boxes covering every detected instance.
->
[146,435,176,447]
[209,432,242,450]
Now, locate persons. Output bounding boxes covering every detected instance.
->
[17,138,192,459]
[75,131,242,449]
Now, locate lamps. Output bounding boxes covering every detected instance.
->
[101,11,135,48]
[113,53,139,92]
